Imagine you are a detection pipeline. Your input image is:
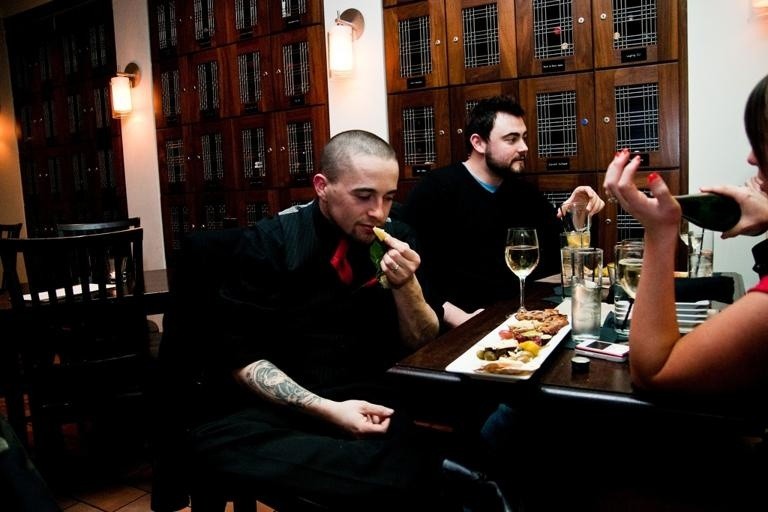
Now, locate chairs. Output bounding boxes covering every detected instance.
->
[8,242,152,460]
[64,219,140,287]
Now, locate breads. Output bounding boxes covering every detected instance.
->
[507,308,569,335]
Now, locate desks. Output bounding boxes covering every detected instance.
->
[3,269,182,403]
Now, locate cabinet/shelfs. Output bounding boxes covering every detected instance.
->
[149,1,330,265]
[3,0,128,238]
[381,0,689,270]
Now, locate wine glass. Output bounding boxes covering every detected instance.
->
[504,227,541,319]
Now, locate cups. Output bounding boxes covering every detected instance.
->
[606,238,646,300]
[558,200,592,250]
[570,247,603,343]
[687,230,715,279]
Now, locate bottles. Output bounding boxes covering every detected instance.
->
[672,193,768,237]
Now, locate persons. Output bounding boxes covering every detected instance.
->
[600,73,767,511]
[390,92,608,344]
[197,129,451,511]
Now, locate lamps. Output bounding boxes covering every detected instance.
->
[328,9,365,75]
[108,63,141,119]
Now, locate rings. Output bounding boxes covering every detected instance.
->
[392,264,399,273]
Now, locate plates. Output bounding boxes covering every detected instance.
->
[612,298,710,343]
[444,310,573,383]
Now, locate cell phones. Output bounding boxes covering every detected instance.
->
[574,339,629,363]
